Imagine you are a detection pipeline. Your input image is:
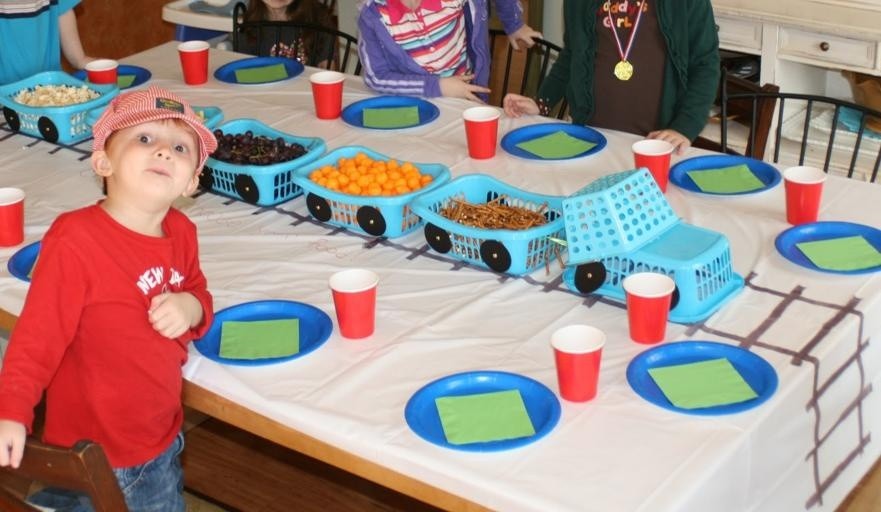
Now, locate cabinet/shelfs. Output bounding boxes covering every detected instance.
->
[695,0,881,183]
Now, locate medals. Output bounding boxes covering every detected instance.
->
[612,61,634,81]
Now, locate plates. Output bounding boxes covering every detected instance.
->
[343,95,443,130]
[672,153,782,196]
[213,55,303,84]
[628,342,783,414]
[192,298,336,364]
[404,371,563,449]
[72,65,152,88]
[779,221,881,277]
[8,241,44,283]
[502,123,608,161]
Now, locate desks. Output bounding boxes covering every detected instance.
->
[0,40,881,510]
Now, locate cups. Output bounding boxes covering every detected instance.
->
[177,40,211,85]
[783,166,826,225]
[552,324,606,402]
[464,105,500,159]
[633,140,673,195]
[327,268,380,340]
[624,272,675,346]
[84,59,120,87]
[308,71,346,119]
[1,186,25,248]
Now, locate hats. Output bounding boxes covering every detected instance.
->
[92,86,218,174]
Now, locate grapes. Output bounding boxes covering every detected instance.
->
[208,129,308,168]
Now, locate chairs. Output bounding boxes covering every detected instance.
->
[718,66,880,184]
[1,439,126,510]
[487,30,572,120]
[233,3,363,76]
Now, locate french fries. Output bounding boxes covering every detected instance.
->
[441,193,567,276]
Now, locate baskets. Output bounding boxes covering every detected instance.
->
[199,118,326,209]
[0,71,121,145]
[562,167,684,264]
[562,222,745,324]
[86,103,223,129]
[294,147,450,237]
[411,174,567,276]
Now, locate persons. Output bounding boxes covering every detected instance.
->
[0,84,218,512]
[504,0,721,156]
[357,1,543,106]
[1,0,97,86]
[235,1,338,71]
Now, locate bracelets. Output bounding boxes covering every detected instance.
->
[532,96,549,117]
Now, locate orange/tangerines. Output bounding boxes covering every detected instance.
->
[308,152,435,232]
